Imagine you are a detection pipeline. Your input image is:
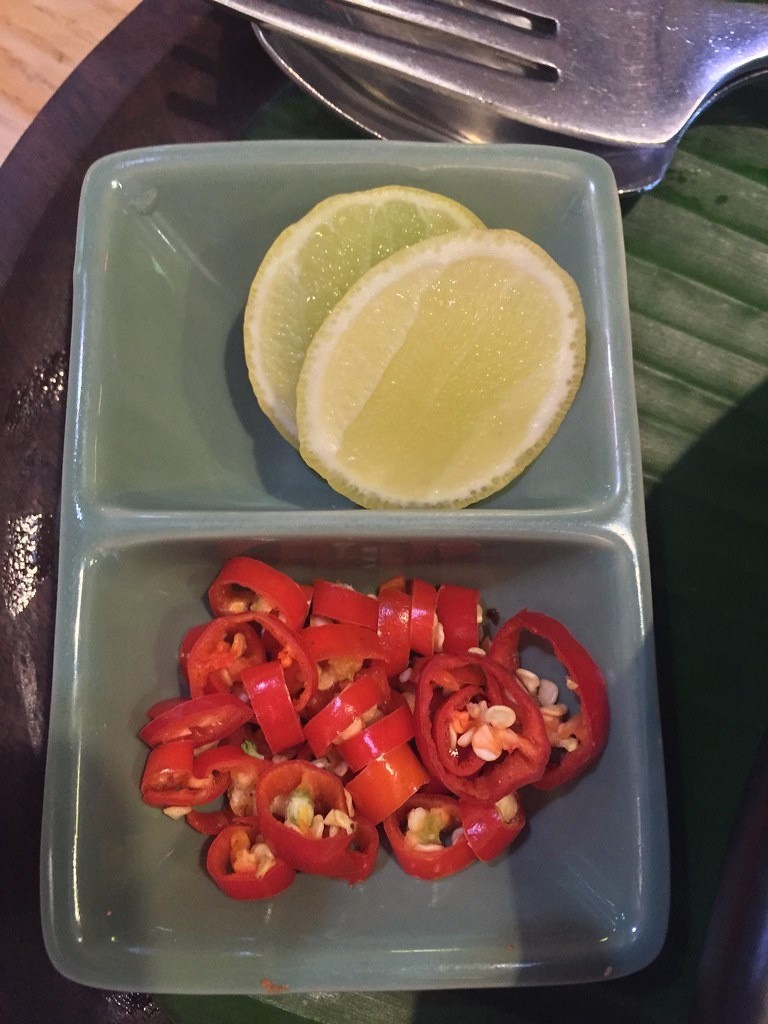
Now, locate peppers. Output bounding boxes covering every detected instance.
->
[140,553,613,896]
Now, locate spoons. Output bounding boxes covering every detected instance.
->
[246,16,766,196]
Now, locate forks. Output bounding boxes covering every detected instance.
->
[212,0,768,144]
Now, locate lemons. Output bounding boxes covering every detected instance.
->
[294,226,587,512]
[240,184,489,448]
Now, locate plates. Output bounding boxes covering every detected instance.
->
[40,137,672,992]
